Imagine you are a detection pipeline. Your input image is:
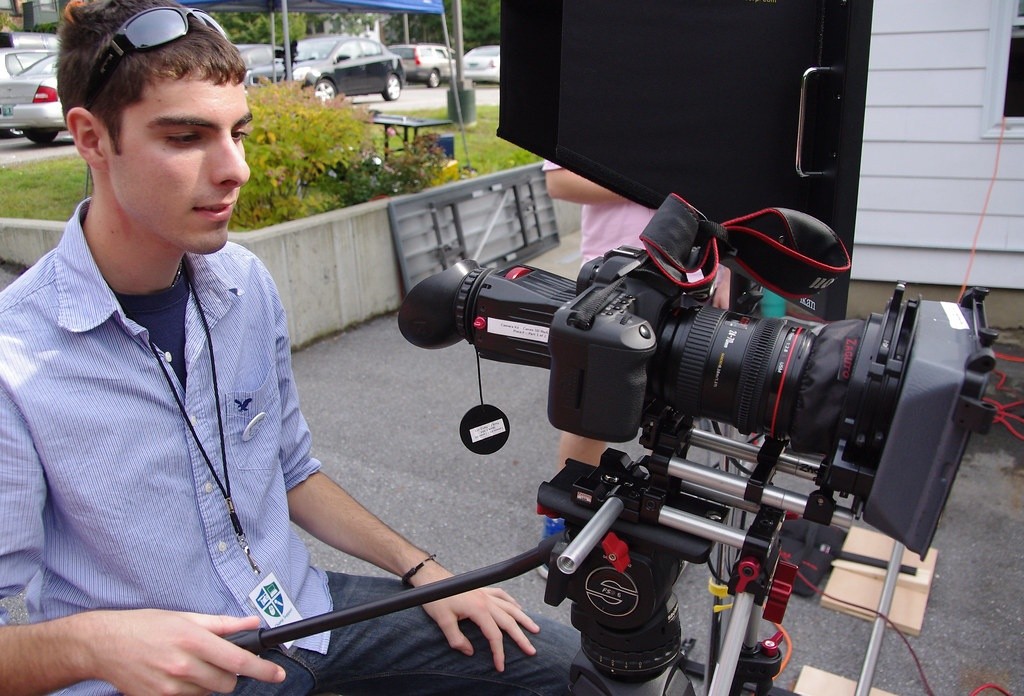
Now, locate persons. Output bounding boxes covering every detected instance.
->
[1,0,585,696]
[535,161,730,581]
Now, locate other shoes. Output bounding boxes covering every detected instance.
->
[538,515,563,579]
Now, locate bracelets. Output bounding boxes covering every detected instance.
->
[402,554,436,586]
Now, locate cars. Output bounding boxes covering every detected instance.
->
[230,44,287,84]
[384,43,458,88]
[463,44,500,83]
[0,55,70,144]
[242,36,407,104]
[1,29,63,51]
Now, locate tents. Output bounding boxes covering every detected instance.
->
[179,0,472,194]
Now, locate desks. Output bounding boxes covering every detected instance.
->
[374,114,454,161]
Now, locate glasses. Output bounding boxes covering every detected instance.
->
[83,6,230,110]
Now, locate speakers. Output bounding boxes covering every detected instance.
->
[493,1,873,272]
